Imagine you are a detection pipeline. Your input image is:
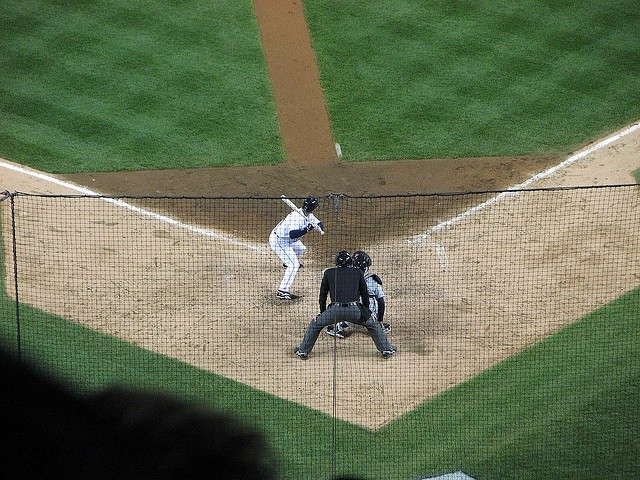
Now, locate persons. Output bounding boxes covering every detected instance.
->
[293,249,398,360]
[268,195,324,300]
[326,250,395,339]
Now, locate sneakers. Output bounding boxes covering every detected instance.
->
[381,346,397,359]
[380,322,391,333]
[283,262,303,268]
[326,326,346,339]
[276,290,296,300]
[293,346,308,360]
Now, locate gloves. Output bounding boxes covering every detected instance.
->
[318,222,323,233]
[305,222,314,232]
[314,311,323,322]
[366,308,372,315]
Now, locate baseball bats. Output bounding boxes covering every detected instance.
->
[279,195,325,236]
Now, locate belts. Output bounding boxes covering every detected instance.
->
[331,301,360,306]
[274,230,280,238]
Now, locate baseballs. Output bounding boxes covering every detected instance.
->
[334,143,342,155]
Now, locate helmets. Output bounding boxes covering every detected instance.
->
[335,250,352,267]
[353,251,372,268]
[303,195,320,212]
